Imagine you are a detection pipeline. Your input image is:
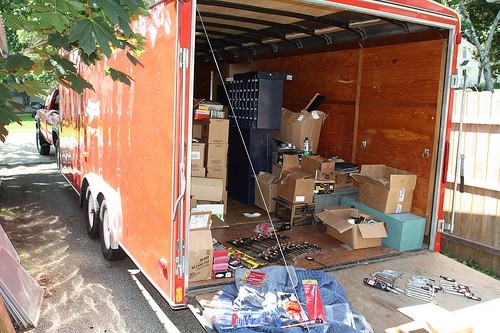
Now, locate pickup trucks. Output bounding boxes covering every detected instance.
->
[30,89,61,170]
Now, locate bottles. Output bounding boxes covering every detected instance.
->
[303,136,311,157]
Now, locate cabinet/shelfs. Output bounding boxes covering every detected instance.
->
[226,127,272,209]
[225,70,287,131]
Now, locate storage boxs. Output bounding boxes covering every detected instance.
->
[188,93,427,283]
